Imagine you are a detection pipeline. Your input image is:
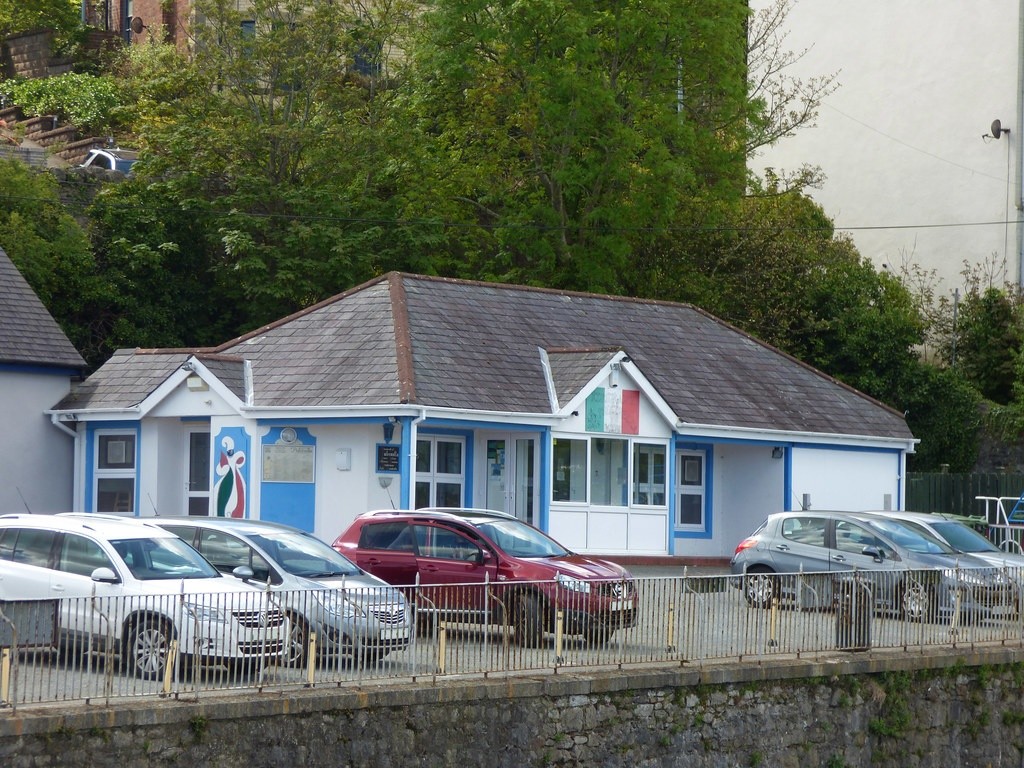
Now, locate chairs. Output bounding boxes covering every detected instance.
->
[102,542,127,560]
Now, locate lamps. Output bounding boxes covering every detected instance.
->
[388,416,401,424]
[182,362,194,371]
[772,447,783,459]
[65,414,78,420]
[130,17,146,34]
[620,356,632,362]
[571,410,579,416]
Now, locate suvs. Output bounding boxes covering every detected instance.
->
[327,505,641,649]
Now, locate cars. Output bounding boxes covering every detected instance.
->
[821,510,1024,608]
[729,509,1017,626]
[0,509,290,682]
[96,516,410,669]
